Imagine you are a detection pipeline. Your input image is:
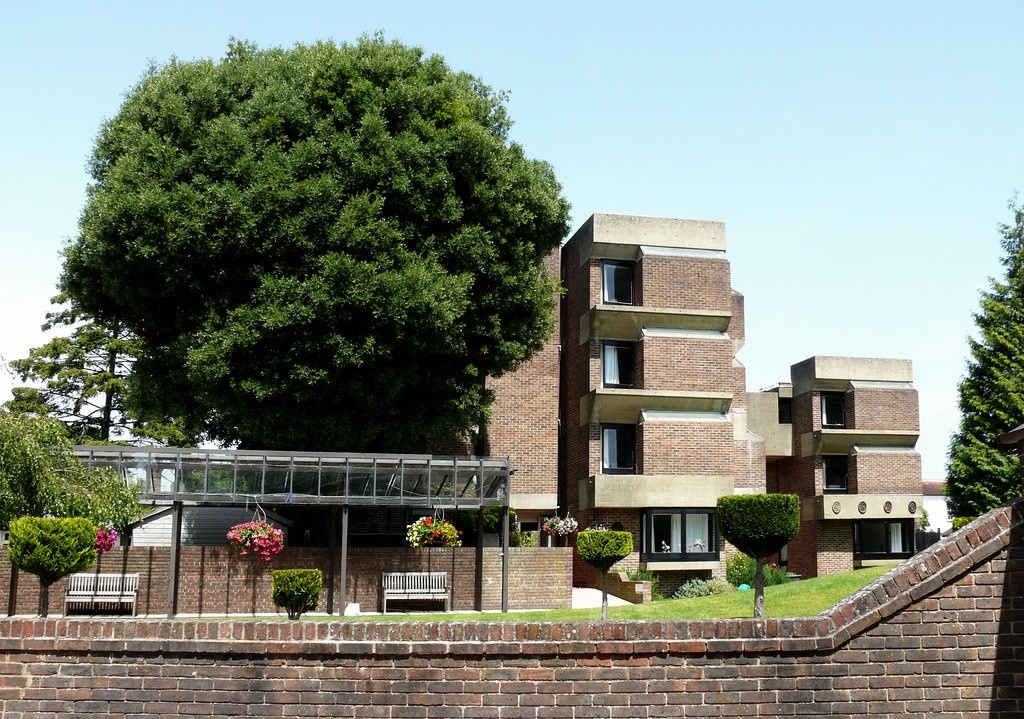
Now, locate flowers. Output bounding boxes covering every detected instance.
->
[541,516,579,538]
[404,514,463,549]
[226,519,286,564]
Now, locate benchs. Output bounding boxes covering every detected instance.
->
[380,571,452,613]
[62,572,140,617]
